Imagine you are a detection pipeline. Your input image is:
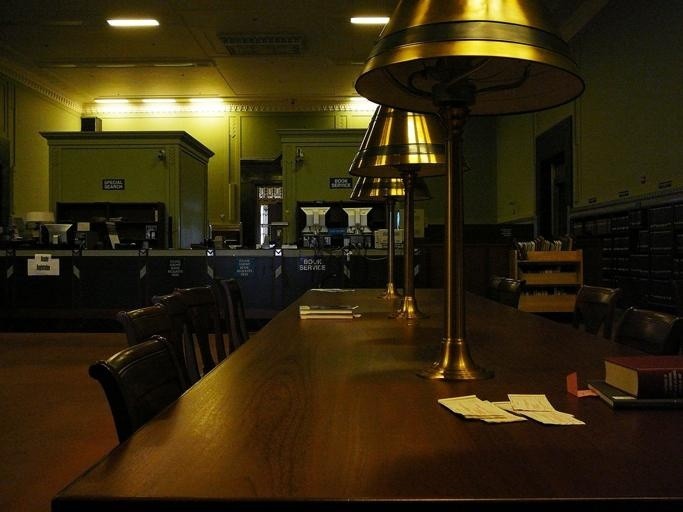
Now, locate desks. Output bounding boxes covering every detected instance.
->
[51,288,683,512]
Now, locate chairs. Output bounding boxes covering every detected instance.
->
[575,284,623,331]
[220,278,250,349]
[610,306,683,356]
[174,285,227,374]
[88,335,184,443]
[151,291,216,388]
[110,303,187,392]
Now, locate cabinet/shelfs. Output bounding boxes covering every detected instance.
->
[56,202,165,250]
[508,249,584,312]
[567,193,683,311]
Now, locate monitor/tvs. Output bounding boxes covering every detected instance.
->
[40,223,76,250]
[108,231,121,250]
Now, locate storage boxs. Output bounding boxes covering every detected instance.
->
[81,118,102,133]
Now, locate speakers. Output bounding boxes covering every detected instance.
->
[81,117,102,131]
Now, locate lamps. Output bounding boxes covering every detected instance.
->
[349,3,586,382]
[350,175,436,300]
[348,102,472,321]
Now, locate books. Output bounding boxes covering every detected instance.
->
[298,303,359,315]
[601,354,682,400]
[298,313,362,321]
[513,233,577,297]
[586,381,682,412]
